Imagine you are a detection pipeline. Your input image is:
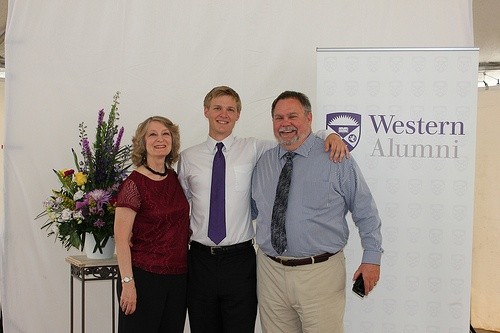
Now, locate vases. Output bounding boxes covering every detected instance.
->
[84,233,115,258]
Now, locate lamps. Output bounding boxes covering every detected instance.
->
[477,62,500,87]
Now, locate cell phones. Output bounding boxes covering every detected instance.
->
[352,273,365,297]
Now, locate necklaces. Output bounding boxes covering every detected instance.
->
[143,162,168,176]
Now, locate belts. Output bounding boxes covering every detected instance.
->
[264,249,341,267]
[190,240,254,256]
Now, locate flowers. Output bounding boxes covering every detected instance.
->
[34,91,134,248]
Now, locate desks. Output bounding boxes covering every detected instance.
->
[64,256,121,333]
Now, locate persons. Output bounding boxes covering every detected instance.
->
[114,116,190,333]
[128,86,350,333]
[251,91,384,333]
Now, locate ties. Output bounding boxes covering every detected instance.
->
[207,142,227,246]
[271,151,298,256]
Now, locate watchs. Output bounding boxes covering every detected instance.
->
[121,275,134,283]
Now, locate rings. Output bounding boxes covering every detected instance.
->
[374,282,377,284]
[123,304,126,307]
